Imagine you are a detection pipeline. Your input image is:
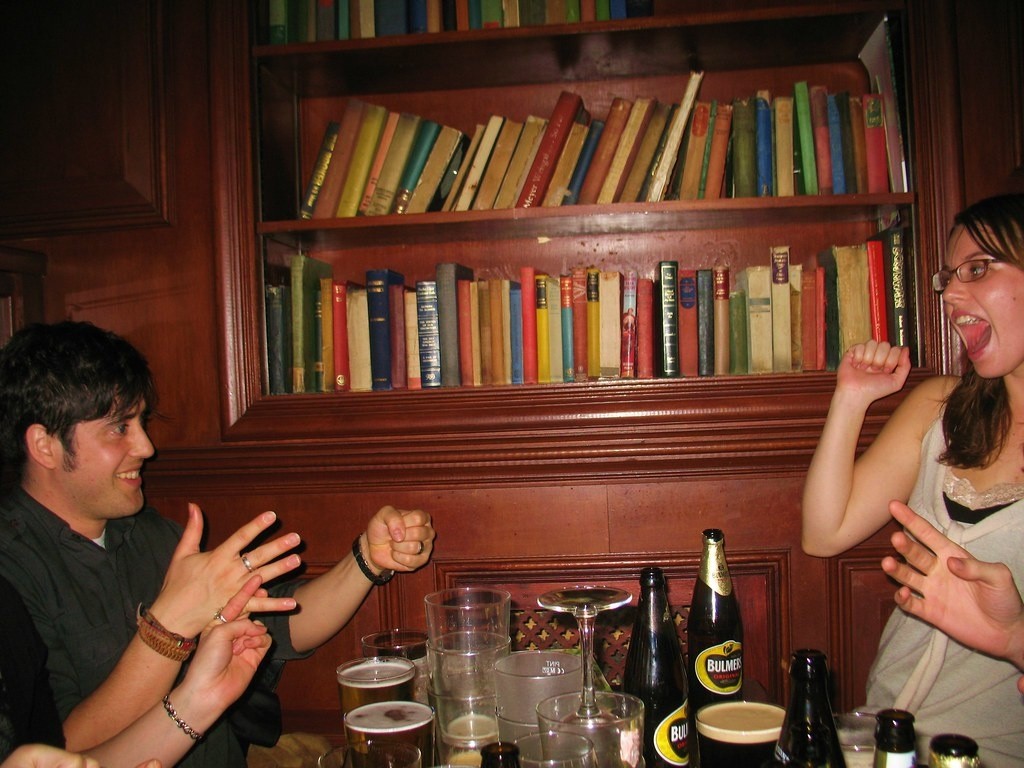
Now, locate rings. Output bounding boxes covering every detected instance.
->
[416,541,424,555]
[242,551,256,573]
[213,606,228,623]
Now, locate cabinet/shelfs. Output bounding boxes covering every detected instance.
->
[0,2,1024,719]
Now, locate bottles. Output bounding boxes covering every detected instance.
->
[480,742,521,768]
[688,528,744,768]
[623,568,688,768]
[874,709,918,768]
[927,733,982,768]
[774,648,848,768]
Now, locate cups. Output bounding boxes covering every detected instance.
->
[536,690,644,768]
[833,711,878,768]
[492,651,585,723]
[317,740,422,768]
[495,712,538,743]
[361,628,429,706]
[695,699,786,768]
[424,587,511,768]
[513,732,599,768]
[336,656,415,713]
[343,700,435,768]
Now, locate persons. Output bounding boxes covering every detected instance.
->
[0,318,436,768]
[802,196,1024,768]
[881,499,1024,698]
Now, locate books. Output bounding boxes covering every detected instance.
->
[563,227,909,384]
[266,0,654,45]
[264,254,561,395]
[298,70,705,220]
[698,13,909,199]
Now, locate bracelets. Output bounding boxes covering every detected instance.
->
[162,694,205,743]
[352,532,395,586]
[135,601,198,662]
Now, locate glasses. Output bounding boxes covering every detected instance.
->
[932,257,1024,291]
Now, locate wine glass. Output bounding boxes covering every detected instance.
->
[539,586,633,725]
